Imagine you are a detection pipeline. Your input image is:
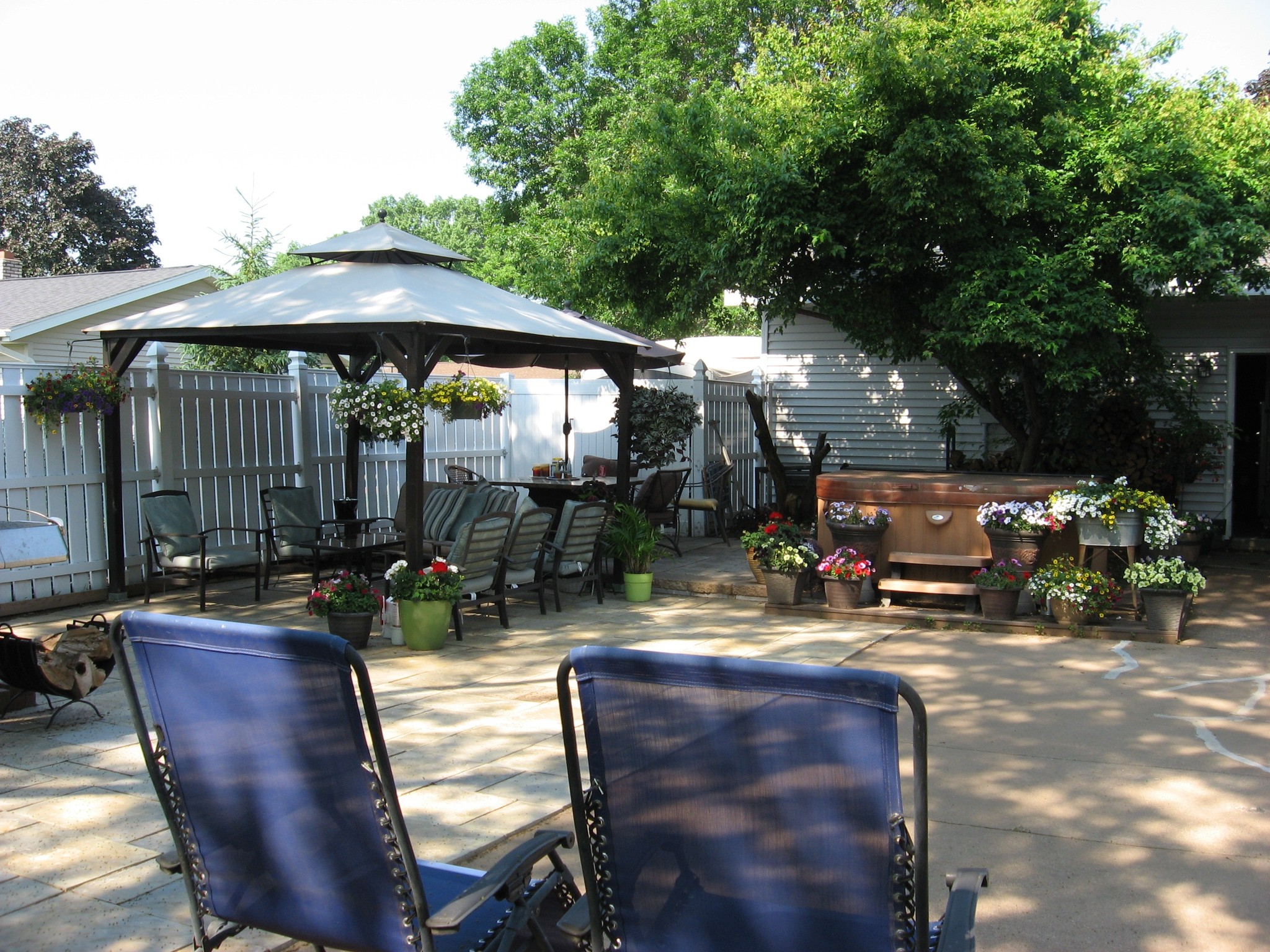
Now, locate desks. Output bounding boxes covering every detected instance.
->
[469,472,643,519]
[299,530,411,586]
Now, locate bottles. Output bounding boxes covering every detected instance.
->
[567,458,571,475]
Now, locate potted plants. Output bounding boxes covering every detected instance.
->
[303,552,464,651]
[745,501,892,608]
[599,504,683,604]
[970,473,1208,637]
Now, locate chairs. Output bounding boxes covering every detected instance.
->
[369,475,609,633]
[553,640,994,952]
[137,489,268,609]
[260,483,374,591]
[109,611,580,952]
[630,467,693,562]
[574,454,641,483]
[443,462,488,488]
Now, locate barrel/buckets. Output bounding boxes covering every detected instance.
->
[333,498,358,521]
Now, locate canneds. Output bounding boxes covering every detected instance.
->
[599,465,606,477]
